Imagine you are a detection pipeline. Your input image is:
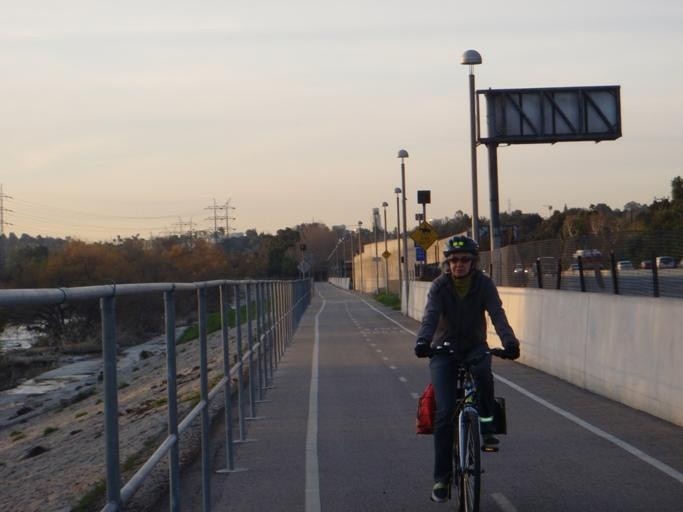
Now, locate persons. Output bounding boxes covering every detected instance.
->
[412,233,523,505]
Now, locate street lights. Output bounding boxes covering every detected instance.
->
[393,186,402,304]
[372,207,380,297]
[356,219,366,298]
[325,227,357,295]
[382,201,390,299]
[458,46,482,273]
[393,147,411,318]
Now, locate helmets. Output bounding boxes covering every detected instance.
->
[442,235,478,255]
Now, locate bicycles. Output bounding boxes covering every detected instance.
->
[412,343,510,512]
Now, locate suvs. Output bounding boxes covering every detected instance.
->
[531,255,559,278]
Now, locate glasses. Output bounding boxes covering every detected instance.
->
[452,256,469,263]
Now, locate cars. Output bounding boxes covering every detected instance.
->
[567,256,683,271]
[512,263,529,277]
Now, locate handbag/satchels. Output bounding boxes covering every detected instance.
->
[416,383,436,434]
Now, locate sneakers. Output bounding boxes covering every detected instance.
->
[482,432,502,448]
[433,481,448,502]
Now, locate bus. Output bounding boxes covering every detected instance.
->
[572,249,603,271]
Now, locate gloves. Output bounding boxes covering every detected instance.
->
[505,341,518,359]
[414,339,430,357]
